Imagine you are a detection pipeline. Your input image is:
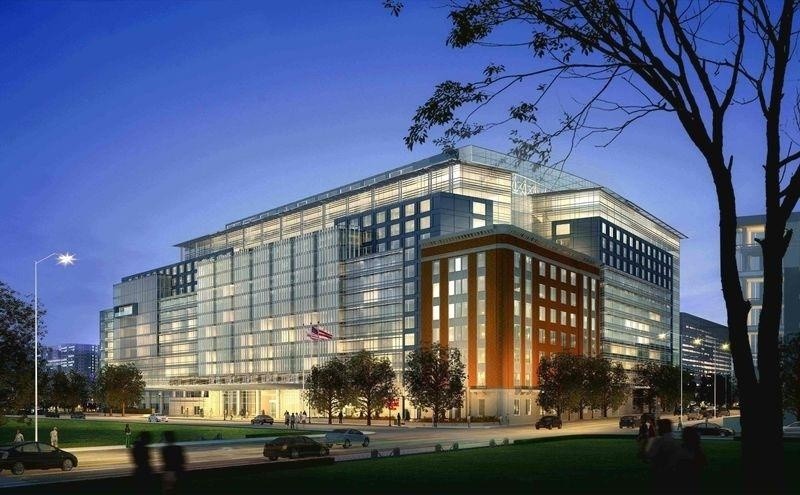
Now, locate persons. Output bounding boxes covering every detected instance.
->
[129,429,154,495]
[633,416,649,464]
[645,418,693,495]
[49,426,59,449]
[466,413,471,429]
[646,415,657,438]
[13,429,25,444]
[283,409,308,429]
[676,424,711,495]
[158,430,188,495]
[338,412,344,424]
[677,416,684,430]
[396,412,401,427]
[124,423,133,448]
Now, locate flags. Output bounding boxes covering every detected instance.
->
[319,328,334,341]
[303,327,319,342]
[310,327,324,340]
